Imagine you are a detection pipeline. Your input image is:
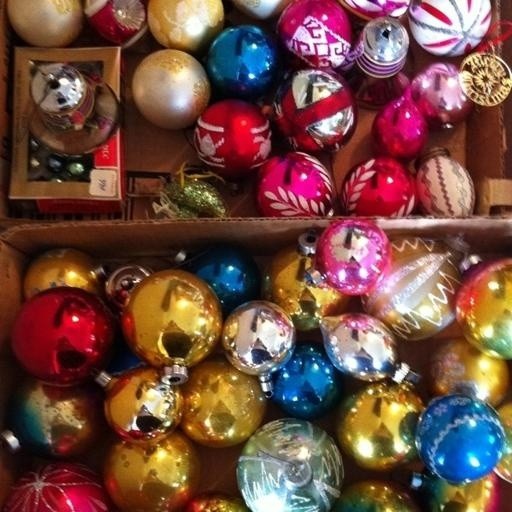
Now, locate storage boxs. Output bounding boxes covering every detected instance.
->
[2,0,512,511]
